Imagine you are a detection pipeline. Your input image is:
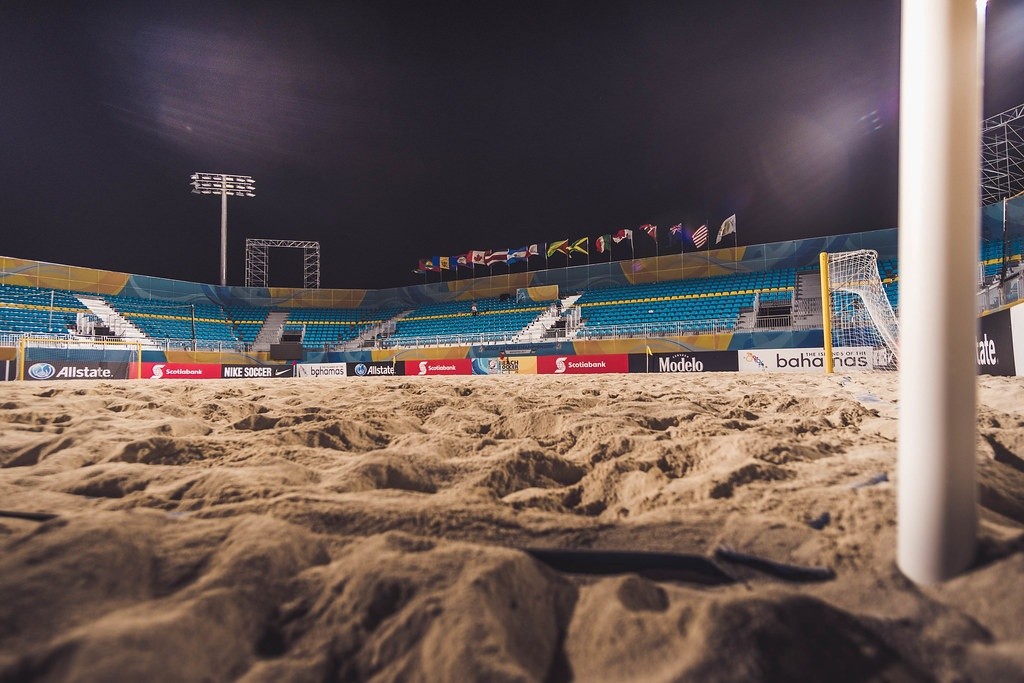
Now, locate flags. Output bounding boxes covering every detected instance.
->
[692,220,708,248]
[639,224,656,239]
[612,229,631,243]
[669,222,682,235]
[415,236,588,274]
[596,234,610,253]
[716,214,736,244]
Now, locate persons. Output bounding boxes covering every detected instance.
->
[472,305,477,315]
[556,298,563,316]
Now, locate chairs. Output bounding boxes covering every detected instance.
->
[0,236,1024,350]
